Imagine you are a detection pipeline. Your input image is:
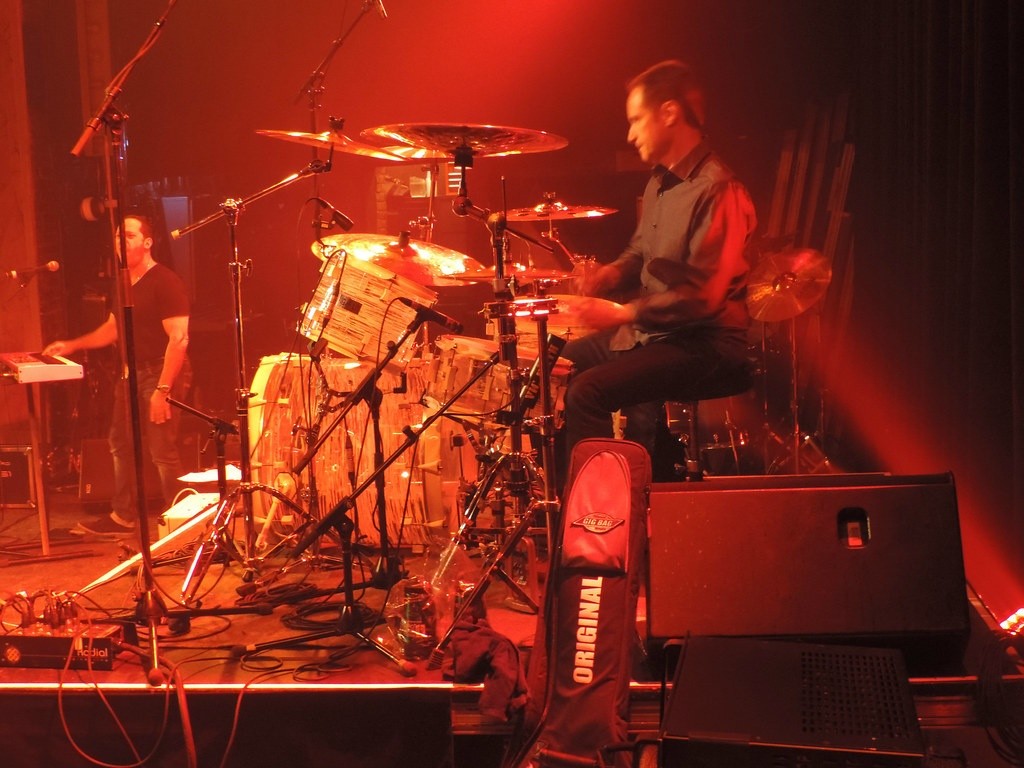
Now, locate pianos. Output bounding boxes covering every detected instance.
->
[0,349,83,556]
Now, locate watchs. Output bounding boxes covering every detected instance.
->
[156,384,174,394]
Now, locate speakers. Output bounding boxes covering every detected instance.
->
[643,471,972,694]
[0,688,454,768]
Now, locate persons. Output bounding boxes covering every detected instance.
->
[43,215,193,535]
[515,60,758,578]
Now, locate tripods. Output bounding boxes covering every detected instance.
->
[70,0,567,684]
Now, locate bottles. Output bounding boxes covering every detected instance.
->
[402,572,487,656]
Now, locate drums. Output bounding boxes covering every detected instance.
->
[425,333,574,433]
[491,295,625,350]
[294,247,439,377]
[248,352,531,553]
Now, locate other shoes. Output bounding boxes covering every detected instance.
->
[78,516,136,539]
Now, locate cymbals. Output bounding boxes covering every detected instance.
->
[437,262,582,288]
[380,146,456,158]
[359,123,569,158]
[488,201,620,221]
[310,233,487,286]
[254,128,408,161]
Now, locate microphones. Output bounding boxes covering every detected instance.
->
[6,261,60,280]
[400,298,464,335]
[373,0,388,20]
[316,198,354,230]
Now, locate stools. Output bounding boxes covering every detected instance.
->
[660,366,756,480]
